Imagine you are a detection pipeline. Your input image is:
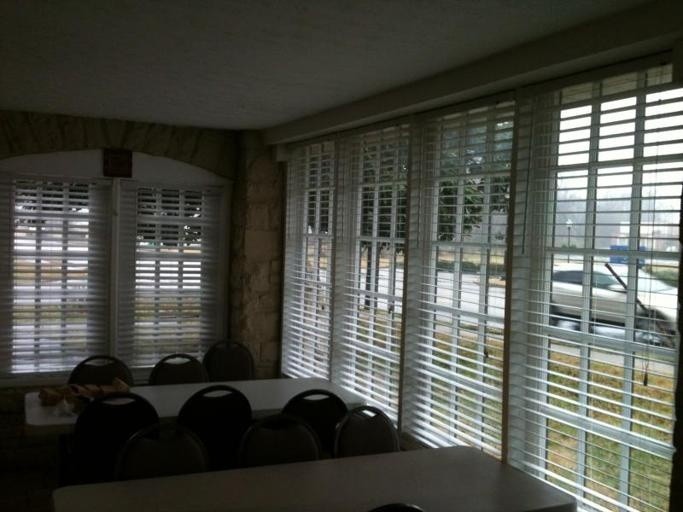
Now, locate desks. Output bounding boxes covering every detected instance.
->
[23,378,366,484]
[50,447,576,511]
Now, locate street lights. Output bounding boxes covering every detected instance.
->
[566,219,573,264]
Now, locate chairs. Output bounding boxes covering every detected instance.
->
[169,386,252,432]
[333,405,398,457]
[208,416,324,469]
[72,390,159,460]
[279,389,345,439]
[67,340,255,384]
[109,423,211,479]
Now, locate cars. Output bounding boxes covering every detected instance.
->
[551,262,678,347]
